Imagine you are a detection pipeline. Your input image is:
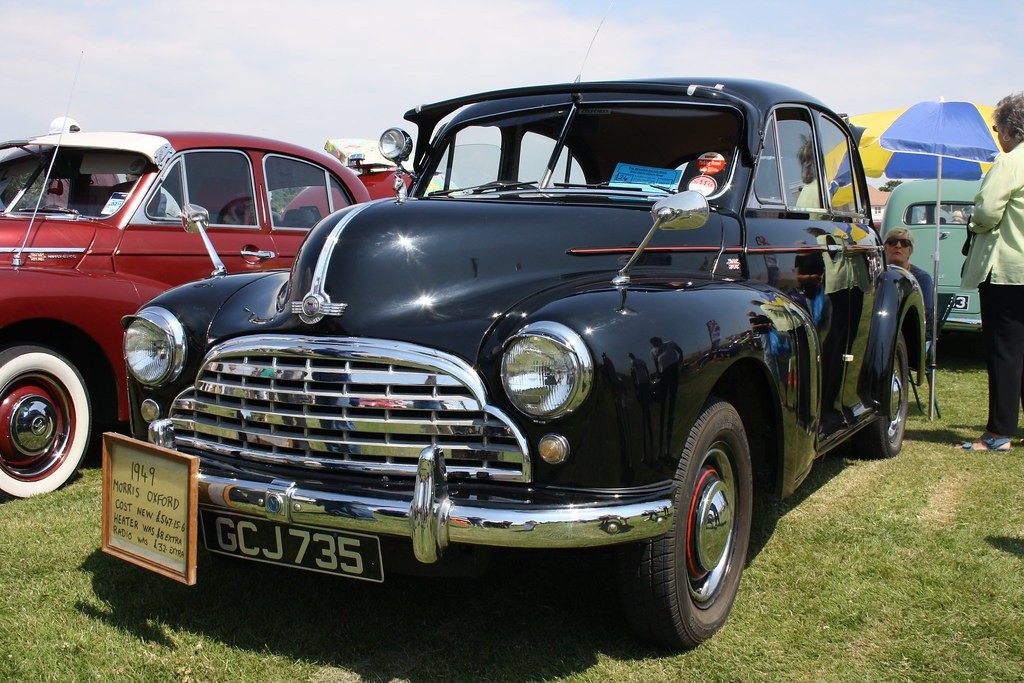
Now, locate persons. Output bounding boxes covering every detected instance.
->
[958,92,1024,451]
[883,228,933,372]
[949,210,963,224]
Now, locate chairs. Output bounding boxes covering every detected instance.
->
[908,293,956,419]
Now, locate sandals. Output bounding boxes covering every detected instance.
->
[954,431,1013,453]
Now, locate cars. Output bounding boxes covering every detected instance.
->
[0,130,461,501]
[880,178,983,334]
[121,77,927,654]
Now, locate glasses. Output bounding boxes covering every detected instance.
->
[992,124,1001,132]
[883,237,913,248]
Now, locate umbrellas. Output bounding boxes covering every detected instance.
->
[848,101,1005,420]
